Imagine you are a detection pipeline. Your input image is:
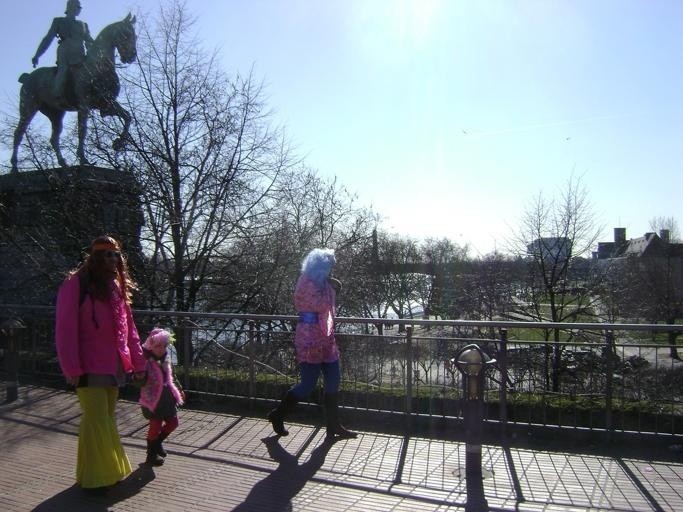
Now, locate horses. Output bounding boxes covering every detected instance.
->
[10,11,137,174]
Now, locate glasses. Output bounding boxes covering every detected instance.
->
[104,250,121,258]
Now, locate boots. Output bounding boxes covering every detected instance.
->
[324,392,357,438]
[267,390,297,436]
[157,431,169,457]
[146,439,164,464]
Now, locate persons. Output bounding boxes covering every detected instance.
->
[31,0,94,98]
[267,246,358,440]
[131,328,185,465]
[54,236,147,489]
